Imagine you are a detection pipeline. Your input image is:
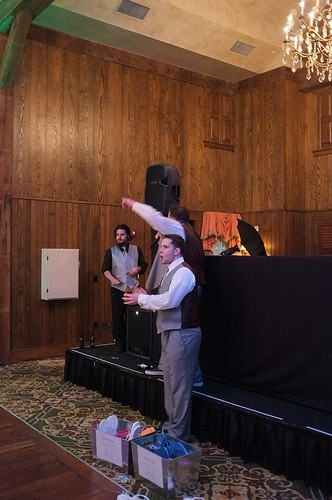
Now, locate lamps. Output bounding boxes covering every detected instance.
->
[281,0,332,83]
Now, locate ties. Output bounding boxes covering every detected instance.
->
[122,246,127,258]
[161,266,169,286]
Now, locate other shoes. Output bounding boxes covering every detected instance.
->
[193,380,203,386]
[116,341,126,352]
[145,366,164,375]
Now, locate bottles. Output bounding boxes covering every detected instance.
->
[90,330,96,349]
[79,331,85,349]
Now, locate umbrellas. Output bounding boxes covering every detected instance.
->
[236,217,267,256]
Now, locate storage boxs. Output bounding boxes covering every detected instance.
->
[90,418,202,497]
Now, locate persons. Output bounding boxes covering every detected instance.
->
[122,234,201,442]
[120,196,204,386]
[102,224,148,343]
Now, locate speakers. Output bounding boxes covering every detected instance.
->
[126,307,161,362]
[145,164,180,218]
